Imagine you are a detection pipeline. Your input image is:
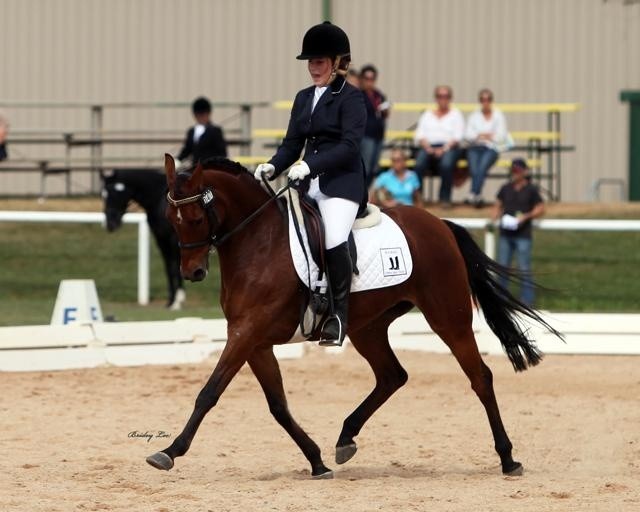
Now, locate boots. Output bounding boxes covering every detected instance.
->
[320,241,353,341]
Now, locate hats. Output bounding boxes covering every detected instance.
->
[512,160,526,170]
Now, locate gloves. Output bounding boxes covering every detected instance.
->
[287,160,310,186]
[254,163,275,181]
[173,157,194,172]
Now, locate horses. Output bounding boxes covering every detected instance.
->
[145,152,567,479]
[98,168,186,309]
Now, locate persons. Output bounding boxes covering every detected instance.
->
[462,88,509,209]
[347,69,360,89]
[413,83,467,210]
[173,94,226,172]
[253,19,370,342]
[368,147,425,208]
[488,156,548,309]
[360,64,391,186]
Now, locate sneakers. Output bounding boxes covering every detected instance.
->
[438,191,482,210]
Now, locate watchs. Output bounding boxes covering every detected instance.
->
[442,144,451,153]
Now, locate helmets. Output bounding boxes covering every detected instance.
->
[296,21,350,59]
[192,98,211,115]
